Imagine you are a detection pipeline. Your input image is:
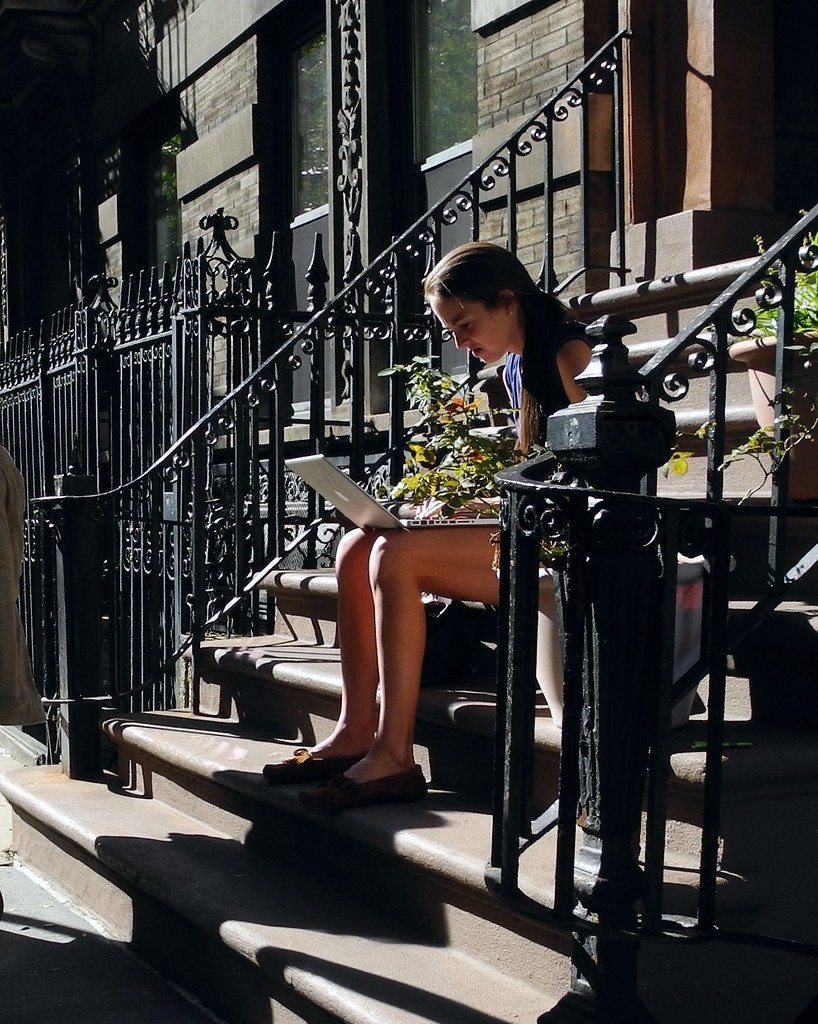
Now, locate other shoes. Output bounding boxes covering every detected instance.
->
[298,764,427,812]
[263,748,368,784]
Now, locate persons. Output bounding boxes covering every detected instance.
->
[263,239,603,816]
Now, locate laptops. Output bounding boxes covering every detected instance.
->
[284,454,501,528]
